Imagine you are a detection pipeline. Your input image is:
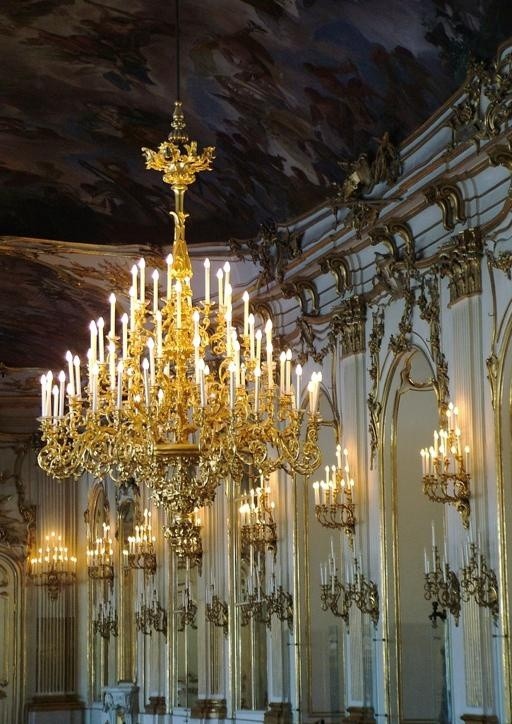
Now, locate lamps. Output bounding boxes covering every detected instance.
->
[418,516,463,632]
[88,520,113,588]
[311,443,359,540]
[420,397,473,530]
[159,505,203,575]
[239,484,279,559]
[126,507,157,577]
[27,527,78,600]
[457,517,503,631]
[30,0,328,549]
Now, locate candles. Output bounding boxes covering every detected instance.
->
[315,535,377,628]
[237,563,292,630]
[170,574,228,632]
[134,577,165,638]
[93,592,118,640]
